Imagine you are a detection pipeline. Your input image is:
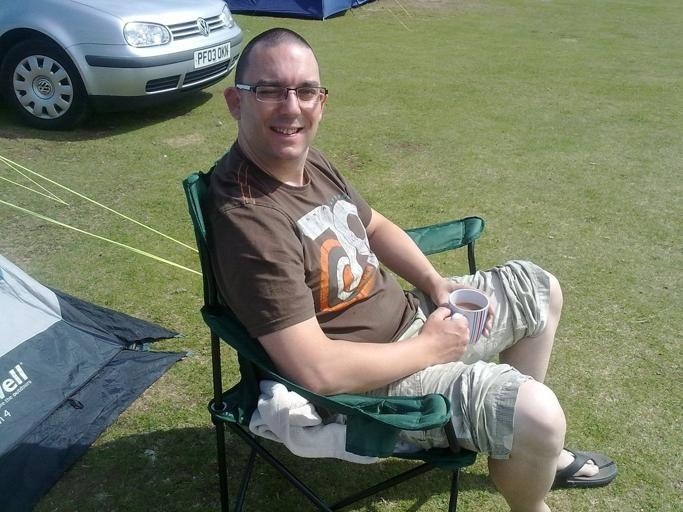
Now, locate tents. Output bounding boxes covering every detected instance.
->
[225,0,378,22]
[0,253,189,512]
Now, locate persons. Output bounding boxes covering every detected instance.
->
[201,26,602,512]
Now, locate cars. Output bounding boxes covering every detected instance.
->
[0,1,245,131]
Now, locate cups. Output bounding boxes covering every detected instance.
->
[449,290,489,344]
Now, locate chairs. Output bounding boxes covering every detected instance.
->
[180,168,487,512]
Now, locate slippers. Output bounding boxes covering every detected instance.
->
[552,446,618,486]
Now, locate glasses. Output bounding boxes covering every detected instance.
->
[233,83,327,109]
